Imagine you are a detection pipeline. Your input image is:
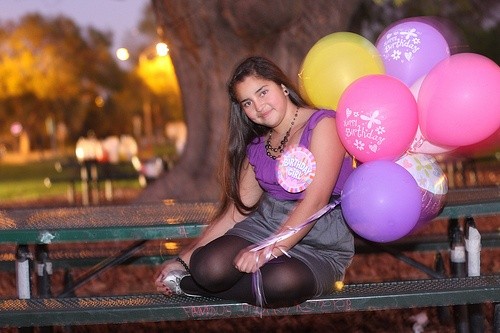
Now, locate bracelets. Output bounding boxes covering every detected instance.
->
[176,257,191,274]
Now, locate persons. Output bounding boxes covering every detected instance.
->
[154,58,355,308]
[75,130,144,178]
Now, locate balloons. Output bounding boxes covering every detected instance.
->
[298,21,500,243]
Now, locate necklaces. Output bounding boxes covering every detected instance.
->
[265,108,298,160]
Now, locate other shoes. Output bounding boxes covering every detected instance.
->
[163,270,203,298]
